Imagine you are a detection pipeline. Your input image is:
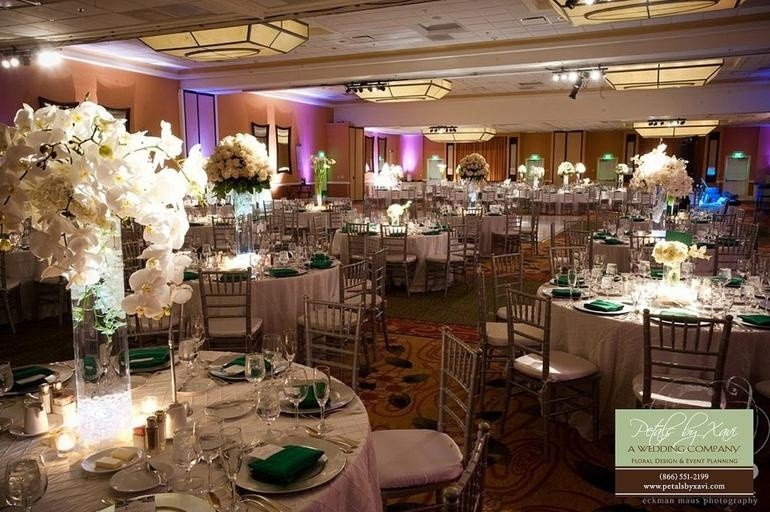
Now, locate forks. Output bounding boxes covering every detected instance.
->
[227,483,285,511]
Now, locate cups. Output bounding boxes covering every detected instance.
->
[24,403,48,433]
[9,414,63,436]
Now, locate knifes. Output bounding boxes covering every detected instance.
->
[201,399,253,408]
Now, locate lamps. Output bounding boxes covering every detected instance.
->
[549,0,746,27]
[422,125,497,144]
[137,19,309,64]
[350,79,452,104]
[633,119,720,138]
[600,57,726,91]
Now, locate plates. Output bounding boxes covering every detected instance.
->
[651,309,711,326]
[205,397,253,418]
[281,379,355,414]
[304,261,336,268]
[109,462,173,492]
[81,446,143,473]
[210,350,286,379]
[272,271,307,276]
[733,312,770,327]
[572,299,630,314]
[85,343,178,377]
[0,364,73,396]
[236,437,347,493]
[175,378,216,396]
[97,493,215,512]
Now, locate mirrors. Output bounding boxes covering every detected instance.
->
[275,124,293,175]
[364,135,375,173]
[705,132,720,178]
[251,121,270,157]
[377,136,387,174]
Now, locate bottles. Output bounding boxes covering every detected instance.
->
[146,417,159,448]
[39,382,50,416]
[52,381,65,399]
[153,410,166,443]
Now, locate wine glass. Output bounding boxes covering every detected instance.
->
[177,338,196,378]
[313,366,331,433]
[193,416,224,496]
[202,244,210,266]
[644,277,657,314]
[6,454,48,512]
[249,250,260,281]
[568,270,576,302]
[592,253,605,273]
[133,427,148,452]
[721,290,734,316]
[697,283,712,312]
[0,361,15,407]
[280,249,289,268]
[639,261,651,278]
[246,353,266,387]
[255,389,280,439]
[713,286,722,308]
[736,258,750,283]
[190,238,201,264]
[606,263,616,277]
[263,334,282,385]
[284,367,308,433]
[216,428,248,512]
[720,267,732,287]
[681,261,695,287]
[629,280,640,321]
[171,427,203,490]
[601,277,613,300]
[282,328,296,371]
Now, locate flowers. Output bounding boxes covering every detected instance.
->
[308,154,336,194]
[573,162,586,174]
[615,163,629,176]
[0,100,210,345]
[202,133,275,201]
[456,152,490,187]
[517,164,527,178]
[529,165,545,178]
[628,143,695,223]
[557,161,575,177]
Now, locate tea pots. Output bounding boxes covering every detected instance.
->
[164,401,188,440]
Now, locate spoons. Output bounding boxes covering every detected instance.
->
[304,424,351,453]
